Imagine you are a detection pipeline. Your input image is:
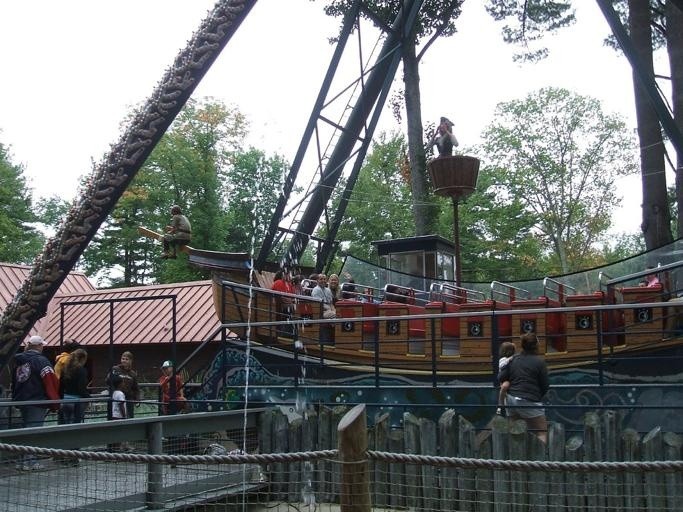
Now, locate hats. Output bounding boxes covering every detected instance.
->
[159,360,172,370]
[27,335,46,347]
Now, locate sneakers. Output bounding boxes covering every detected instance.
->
[12,457,82,471]
[496,408,502,415]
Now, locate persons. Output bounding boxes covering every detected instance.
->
[639,266,659,288]
[495,333,550,445]
[272,270,384,328]
[158,205,191,259]
[159,360,192,414]
[427,116,459,156]
[105,351,140,453]
[9,336,89,470]
